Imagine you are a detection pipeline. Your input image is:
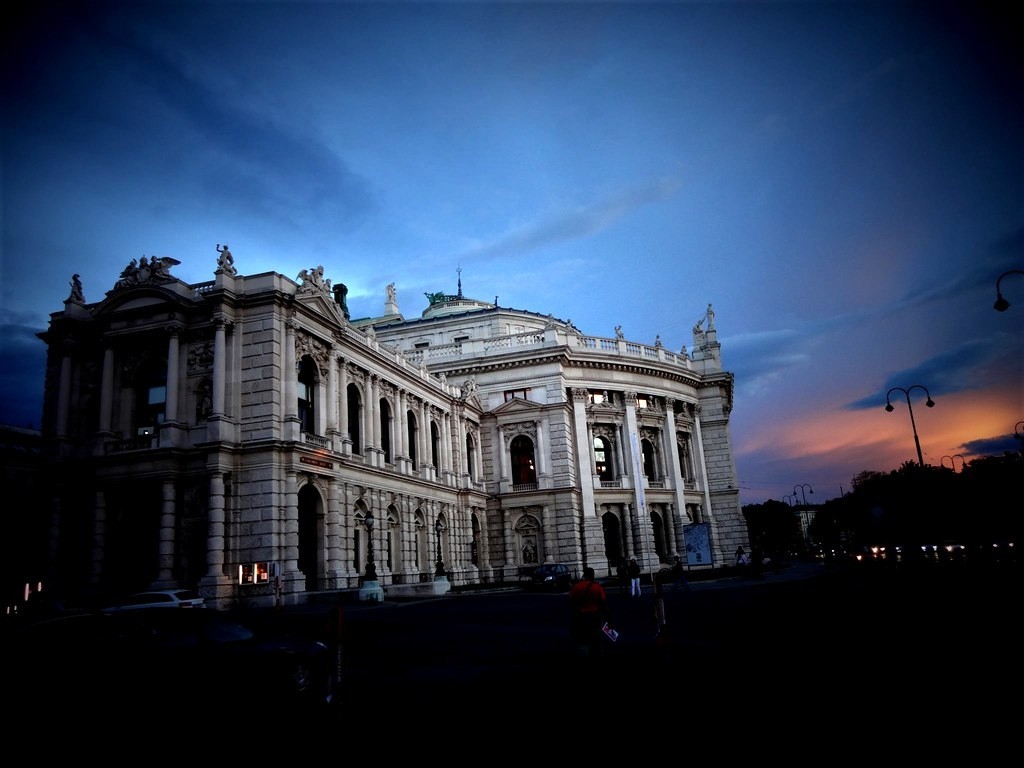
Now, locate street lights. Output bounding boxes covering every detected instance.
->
[363,511,377,581]
[433,519,446,575]
[783,483,814,528]
[884,384,935,493]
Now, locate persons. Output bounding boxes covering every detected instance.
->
[672,556,686,589]
[568,569,614,652]
[735,546,804,578]
[617,558,644,598]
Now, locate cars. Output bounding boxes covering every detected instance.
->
[102,589,205,615]
[520,564,571,592]
